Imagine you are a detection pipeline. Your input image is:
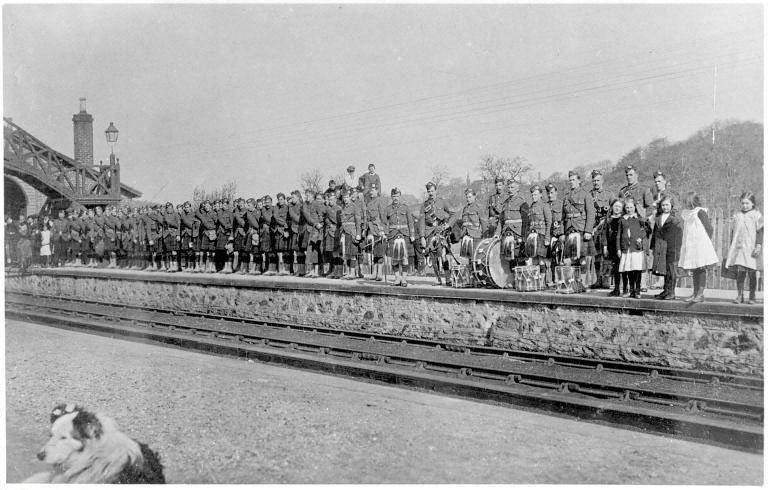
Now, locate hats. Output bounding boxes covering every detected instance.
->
[328,184,401,198]
[567,164,665,180]
[425,181,435,189]
[276,188,316,197]
[494,177,555,193]
[199,196,272,205]
[60,200,191,213]
[464,187,473,195]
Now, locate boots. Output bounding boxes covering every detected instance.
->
[185,261,364,280]
[64,256,179,272]
[368,263,408,287]
[590,261,610,289]
[433,260,452,286]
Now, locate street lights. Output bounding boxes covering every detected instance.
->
[104,120,119,197]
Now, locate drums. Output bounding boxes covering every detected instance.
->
[449,264,472,287]
[474,235,514,289]
[514,266,543,292]
[554,266,586,293]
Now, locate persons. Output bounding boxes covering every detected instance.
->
[5,164,385,272]
[678,192,718,302]
[460,165,684,300]
[383,188,416,288]
[419,181,455,286]
[726,192,763,303]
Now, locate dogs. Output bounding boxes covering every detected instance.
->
[21,402,166,484]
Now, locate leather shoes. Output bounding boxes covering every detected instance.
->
[733,297,756,304]
[607,293,706,302]
